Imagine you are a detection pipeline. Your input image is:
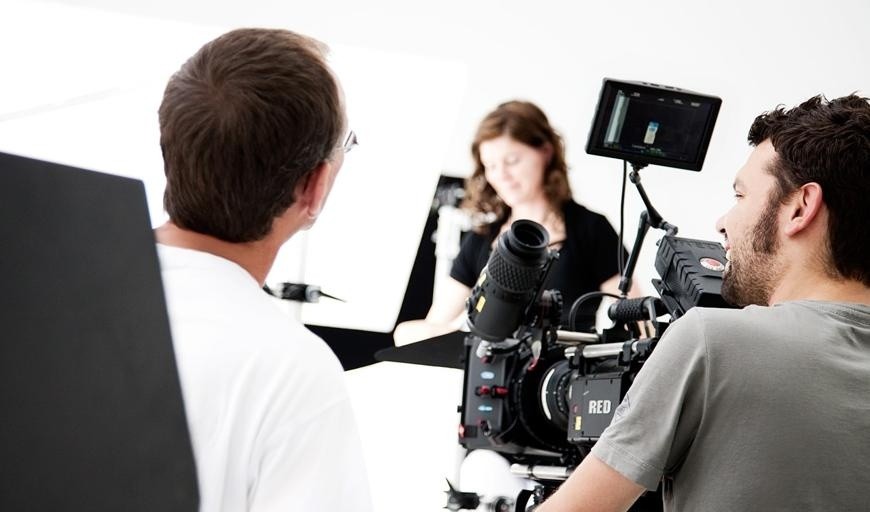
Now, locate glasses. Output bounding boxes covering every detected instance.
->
[304,127,357,194]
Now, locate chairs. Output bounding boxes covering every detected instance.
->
[1,152,199,512]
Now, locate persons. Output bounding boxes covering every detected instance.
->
[531,93,870,512]
[152,26,372,512]
[391,99,657,348]
